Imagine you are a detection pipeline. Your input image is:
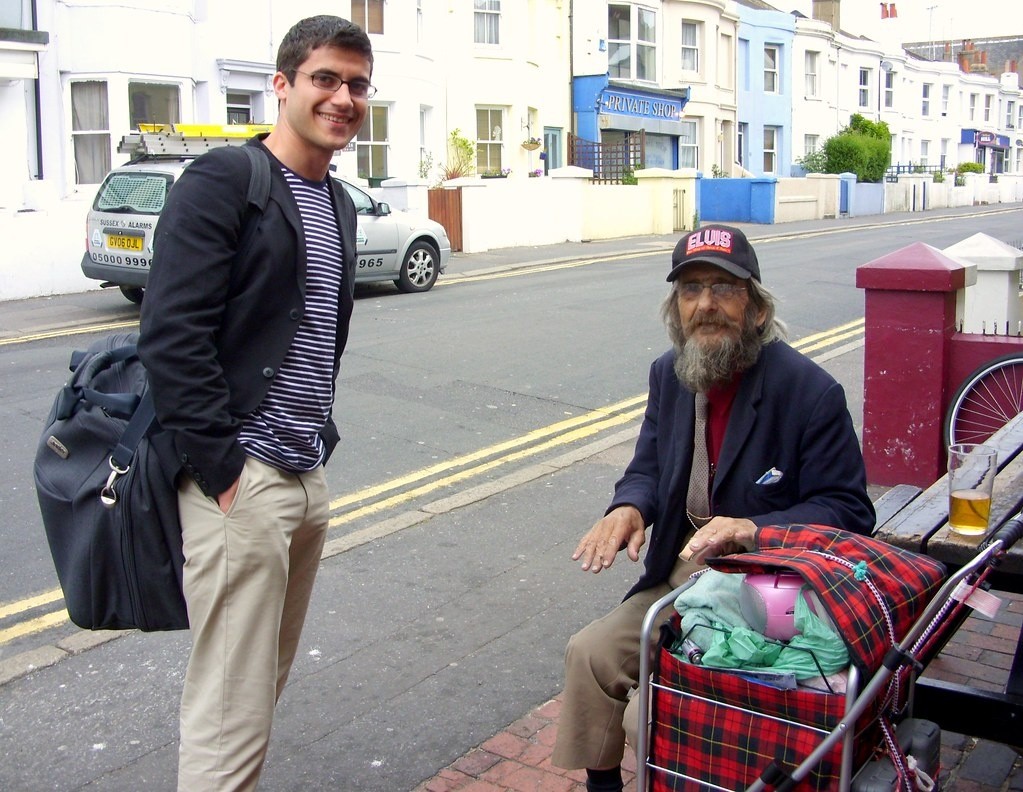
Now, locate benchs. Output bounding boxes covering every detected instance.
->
[872,484,922,537]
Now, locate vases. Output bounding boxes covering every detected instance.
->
[481,176,507,179]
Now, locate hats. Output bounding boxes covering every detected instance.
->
[666,225,761,283]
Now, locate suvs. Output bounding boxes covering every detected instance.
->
[79,122,452,305]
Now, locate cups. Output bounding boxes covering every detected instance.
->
[945,443,997,536]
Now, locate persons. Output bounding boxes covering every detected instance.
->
[132,14,375,792]
[553,222,876,792]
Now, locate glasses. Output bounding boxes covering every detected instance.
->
[290,67,377,99]
[675,282,747,300]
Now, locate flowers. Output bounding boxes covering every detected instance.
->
[523,137,541,145]
[482,168,510,176]
[529,169,543,177]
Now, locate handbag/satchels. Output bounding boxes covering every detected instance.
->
[33,333,190,632]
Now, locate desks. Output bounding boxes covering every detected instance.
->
[878,411,1022,760]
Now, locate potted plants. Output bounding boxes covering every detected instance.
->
[540,148,548,159]
